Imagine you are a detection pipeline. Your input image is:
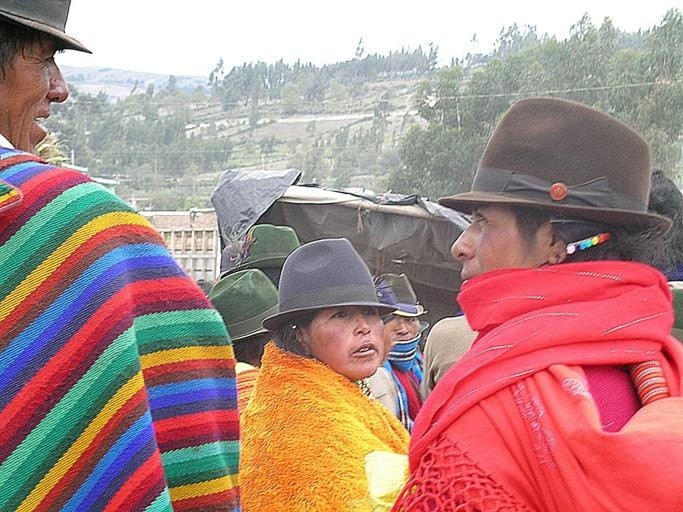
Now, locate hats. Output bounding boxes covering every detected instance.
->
[438,97,675,237]
[208,225,431,342]
[0,0,95,56]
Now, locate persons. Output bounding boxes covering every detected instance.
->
[232,223,300,287]
[355,281,403,424]
[418,313,478,405]
[237,235,410,512]
[633,169,682,344]
[0,0,241,511]
[207,269,278,417]
[391,95,683,512]
[373,273,430,432]
